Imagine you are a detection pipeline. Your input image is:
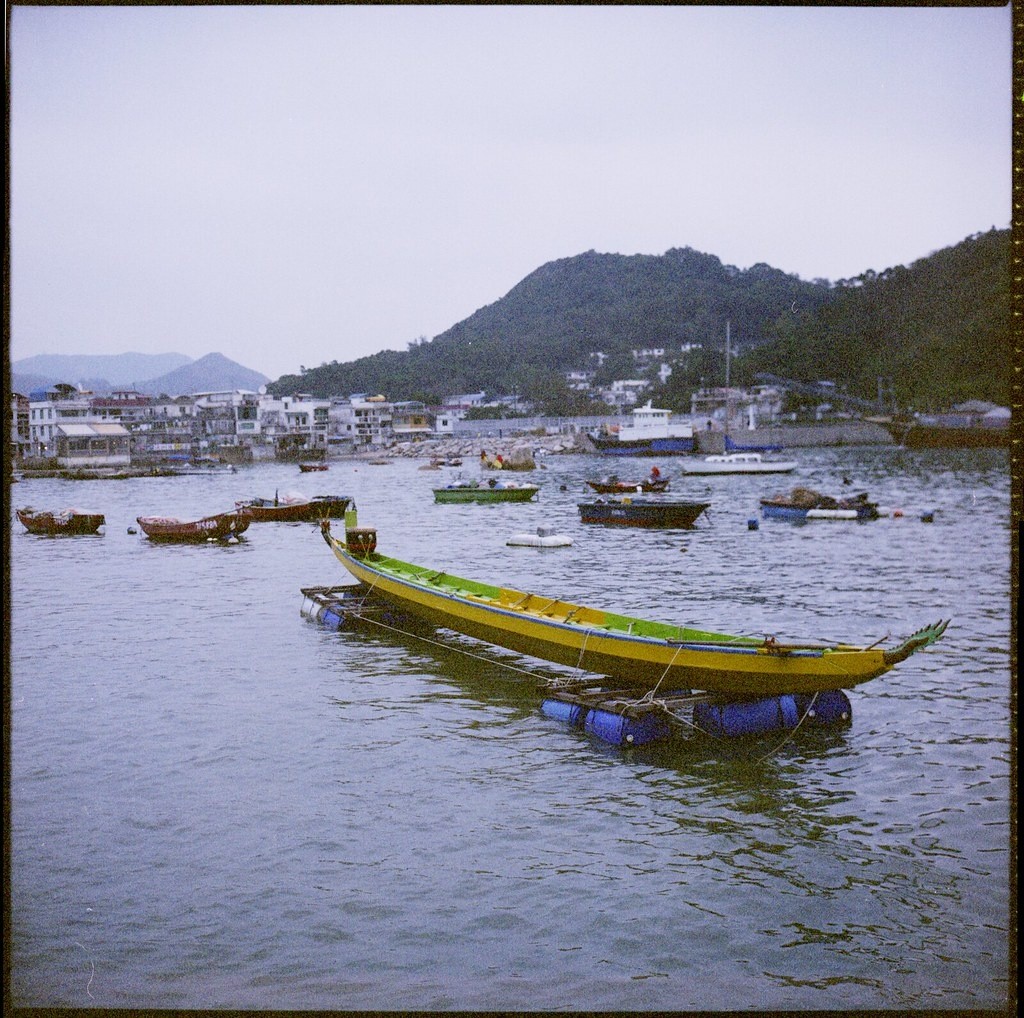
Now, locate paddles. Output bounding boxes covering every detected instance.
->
[538,599,559,614]
[414,573,423,584]
[513,592,532,608]
[860,631,892,652]
[666,635,889,651]
[627,621,635,634]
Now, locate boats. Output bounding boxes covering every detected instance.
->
[576,499,708,524]
[316,499,953,697]
[862,412,1009,448]
[673,450,799,476]
[136,508,252,538]
[22,469,129,481]
[760,502,891,520]
[584,397,694,456]
[299,461,328,473]
[160,465,236,475]
[586,473,678,495]
[14,508,108,536]
[234,496,356,521]
[430,477,540,504]
[505,534,574,546]
[723,434,786,454]
